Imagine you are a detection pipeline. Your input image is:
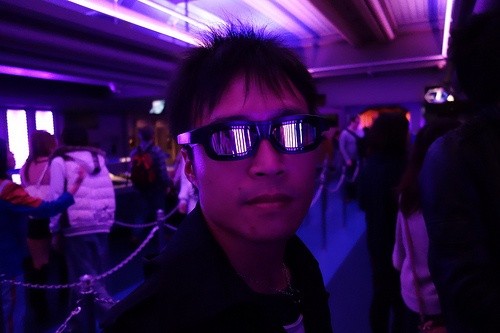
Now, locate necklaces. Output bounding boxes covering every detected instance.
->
[240,260,295,297]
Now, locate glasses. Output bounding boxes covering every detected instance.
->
[177,113,331,161]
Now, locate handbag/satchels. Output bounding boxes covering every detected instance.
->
[49,209,69,239]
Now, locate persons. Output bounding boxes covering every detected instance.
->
[339,1,499,333]
[100,19,333,333]
[0,122,196,333]
